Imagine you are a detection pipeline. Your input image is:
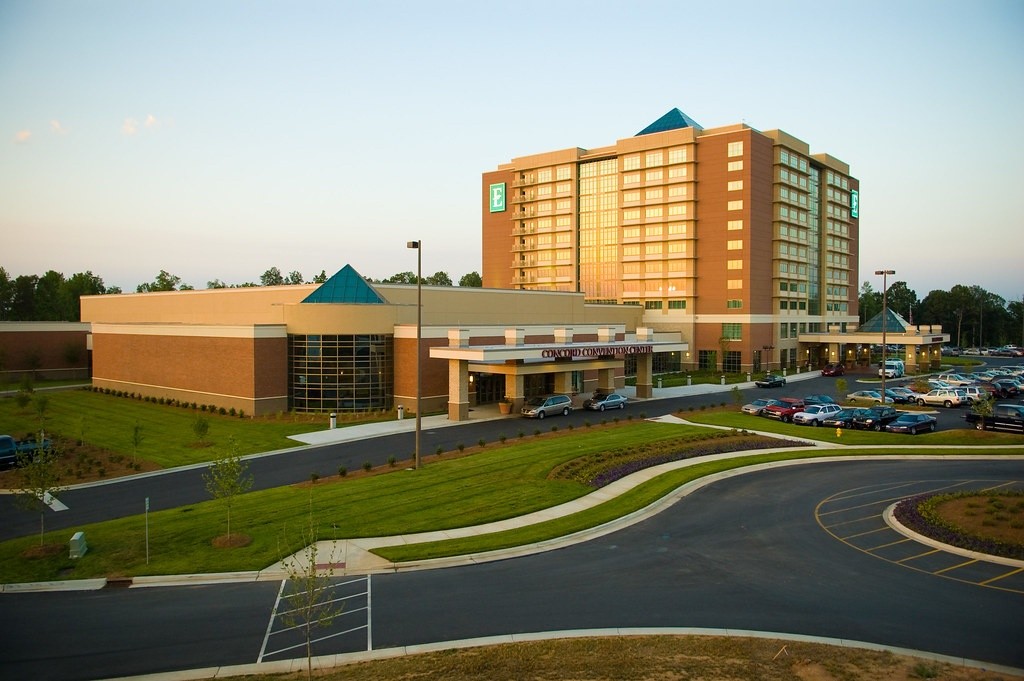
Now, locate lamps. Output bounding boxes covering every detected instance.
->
[469,374,473,383]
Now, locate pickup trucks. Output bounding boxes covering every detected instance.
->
[852,406,909,433]
[0,435,50,472]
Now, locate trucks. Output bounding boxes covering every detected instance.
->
[878,358,904,379]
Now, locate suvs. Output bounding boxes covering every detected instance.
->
[521,394,572,420]
[763,397,806,424]
[792,403,843,429]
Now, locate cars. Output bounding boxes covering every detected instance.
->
[584,393,628,412]
[871,388,907,404]
[801,394,835,405]
[822,408,870,429]
[878,357,902,367]
[821,361,845,377]
[890,386,920,403]
[871,343,1024,360]
[965,402,1024,432]
[906,363,1024,410]
[884,412,937,435]
[846,389,895,406]
[740,398,779,417]
[755,374,787,388]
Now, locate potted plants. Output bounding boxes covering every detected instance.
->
[499,398,512,414]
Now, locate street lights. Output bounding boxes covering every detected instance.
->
[405,239,422,470]
[874,270,895,407]
[762,344,775,369]
